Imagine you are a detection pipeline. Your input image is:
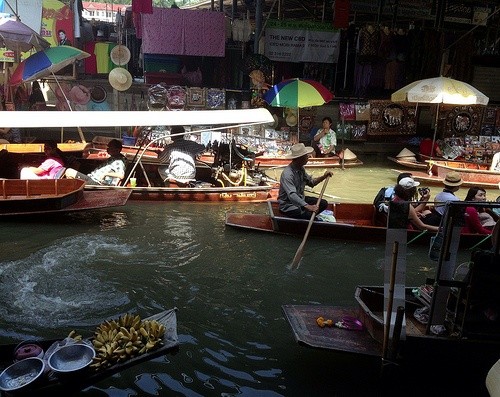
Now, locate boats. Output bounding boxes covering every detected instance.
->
[0,99,500,397]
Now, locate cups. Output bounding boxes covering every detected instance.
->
[130,178,137,187]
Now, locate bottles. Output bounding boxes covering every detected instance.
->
[327,133,331,144]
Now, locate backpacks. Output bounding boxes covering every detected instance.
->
[372,187,390,211]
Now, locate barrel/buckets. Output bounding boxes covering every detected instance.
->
[12,338,44,364]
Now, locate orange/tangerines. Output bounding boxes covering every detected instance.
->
[317,317,332,327]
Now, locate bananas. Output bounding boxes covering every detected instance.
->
[68,312,166,370]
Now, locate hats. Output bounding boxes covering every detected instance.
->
[340,148,357,160]
[443,172,462,186]
[398,177,420,189]
[396,147,416,157]
[109,69,133,91]
[286,114,297,126]
[57,85,68,102]
[286,143,314,159]
[300,117,312,130]
[89,86,107,103]
[72,84,90,104]
[111,45,131,65]
[268,114,278,129]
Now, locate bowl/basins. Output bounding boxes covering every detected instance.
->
[48,344,96,375]
[0,357,45,393]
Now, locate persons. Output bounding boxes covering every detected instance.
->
[277,143,334,221]
[434,171,464,215]
[461,187,493,233]
[57,29,72,47]
[375,173,443,231]
[313,117,336,158]
[19,140,66,180]
[260,77,335,143]
[157,126,205,184]
[65,140,129,185]
[420,130,444,161]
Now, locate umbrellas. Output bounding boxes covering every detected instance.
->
[1,20,51,53]
[9,46,91,128]
[391,75,489,157]
